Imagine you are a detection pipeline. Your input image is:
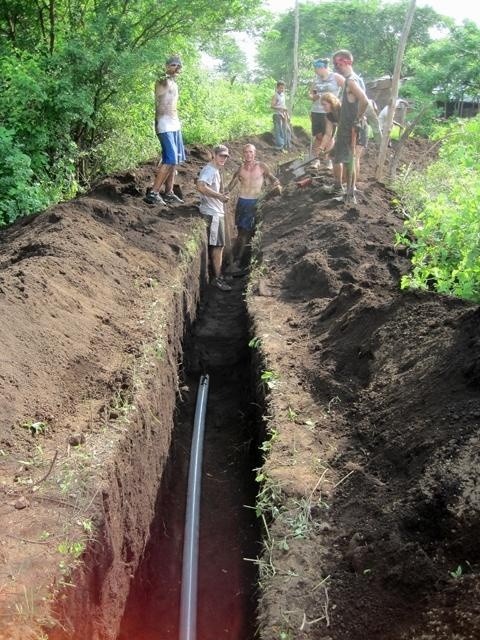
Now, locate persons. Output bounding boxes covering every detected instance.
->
[145,56,186,205]
[196,145,233,291]
[378,99,409,148]
[309,59,346,169]
[359,99,382,149]
[315,93,368,193]
[328,51,369,209]
[224,144,280,277]
[272,80,290,155]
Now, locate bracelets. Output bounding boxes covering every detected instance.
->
[318,147,324,152]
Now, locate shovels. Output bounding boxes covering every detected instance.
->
[280,148,333,180]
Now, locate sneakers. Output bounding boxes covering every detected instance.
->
[146,189,167,207]
[163,191,186,204]
[211,274,233,293]
[321,184,359,207]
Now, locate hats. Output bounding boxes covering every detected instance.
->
[213,144,229,157]
[166,56,182,67]
